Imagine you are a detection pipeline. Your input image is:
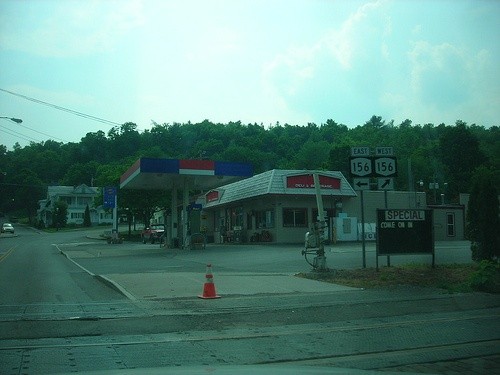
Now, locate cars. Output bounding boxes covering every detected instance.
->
[1,223,15,234]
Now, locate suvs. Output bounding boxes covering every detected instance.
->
[140,223,165,244]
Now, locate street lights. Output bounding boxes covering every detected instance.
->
[0,116,22,124]
[0,172,7,176]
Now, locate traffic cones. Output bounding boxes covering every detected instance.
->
[197,264,223,299]
